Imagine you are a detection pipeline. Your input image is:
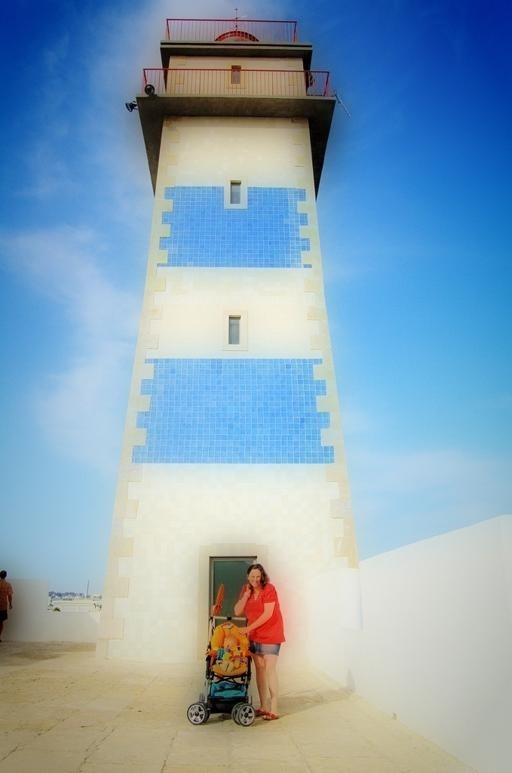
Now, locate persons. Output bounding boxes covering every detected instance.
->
[0,570,12,642]
[234,563,285,720]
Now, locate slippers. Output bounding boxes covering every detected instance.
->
[262,711,280,720]
[254,707,269,717]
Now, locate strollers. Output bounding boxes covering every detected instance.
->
[184,613,258,726]
